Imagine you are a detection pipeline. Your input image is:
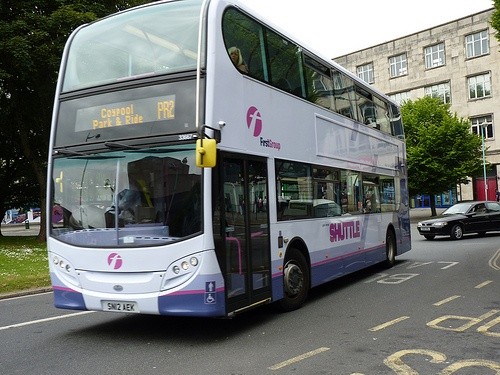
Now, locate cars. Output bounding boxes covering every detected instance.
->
[416,199,500,241]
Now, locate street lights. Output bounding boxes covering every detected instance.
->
[479,121,491,202]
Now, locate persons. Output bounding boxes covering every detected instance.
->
[228,46,246,72]
[106,169,143,222]
[495,189,500,201]
[224,193,267,215]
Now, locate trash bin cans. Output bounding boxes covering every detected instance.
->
[25,220,29,229]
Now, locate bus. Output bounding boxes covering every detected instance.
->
[45,0,413,323]
